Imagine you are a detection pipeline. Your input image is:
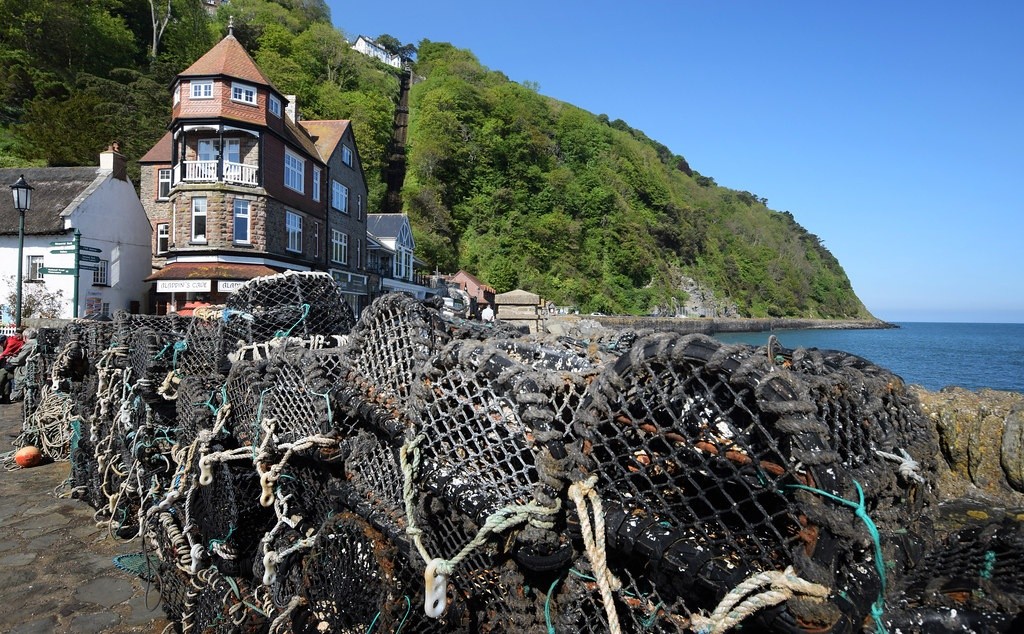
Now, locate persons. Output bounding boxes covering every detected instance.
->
[0,327,38,403]
[481,304,495,323]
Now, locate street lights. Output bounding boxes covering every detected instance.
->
[9,174,35,327]
